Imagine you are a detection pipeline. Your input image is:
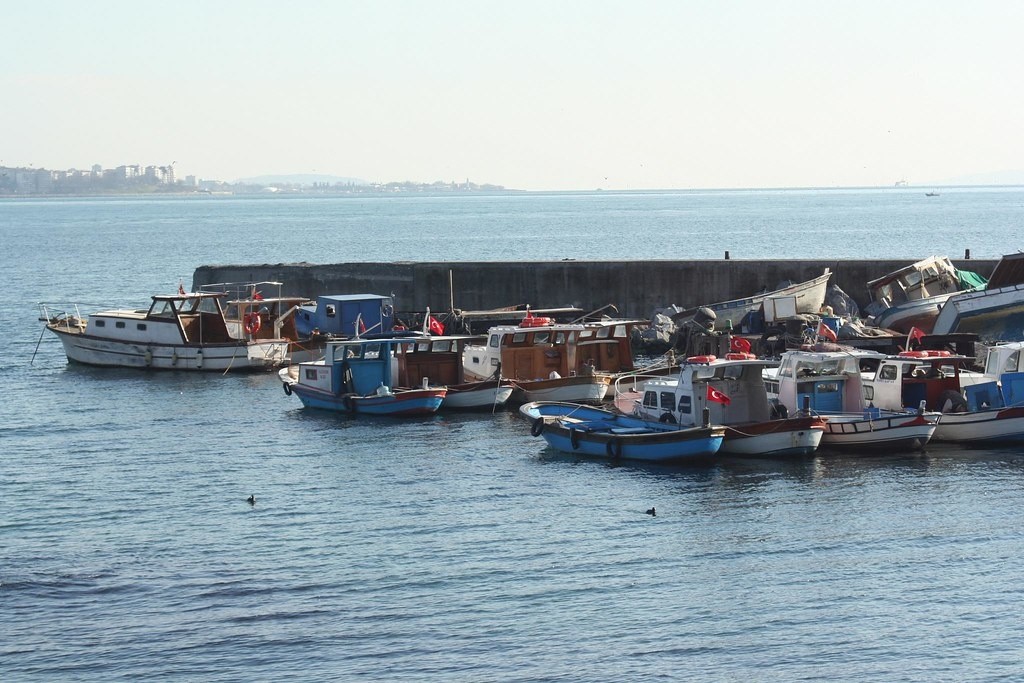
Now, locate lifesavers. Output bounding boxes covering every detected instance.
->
[723,352,756,360]
[800,343,837,351]
[686,354,717,363]
[342,396,355,413]
[518,316,551,328]
[606,436,621,459]
[926,350,949,356]
[283,382,292,396]
[898,350,929,357]
[530,416,545,437]
[244,311,262,334]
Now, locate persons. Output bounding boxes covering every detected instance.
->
[309,327,320,340]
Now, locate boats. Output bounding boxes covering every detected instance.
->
[615,352,831,463]
[393,255,1024,447]
[36,281,311,373]
[277,338,448,420]
[290,292,429,340]
[384,335,515,413]
[725,343,943,452]
[519,399,727,467]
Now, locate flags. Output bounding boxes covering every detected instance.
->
[430,315,445,335]
[707,384,730,403]
[360,318,366,333]
[815,319,837,342]
[179,283,185,294]
[730,334,751,353]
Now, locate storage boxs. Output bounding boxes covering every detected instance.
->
[1000,372,1024,406]
[821,317,840,337]
[964,381,1002,412]
